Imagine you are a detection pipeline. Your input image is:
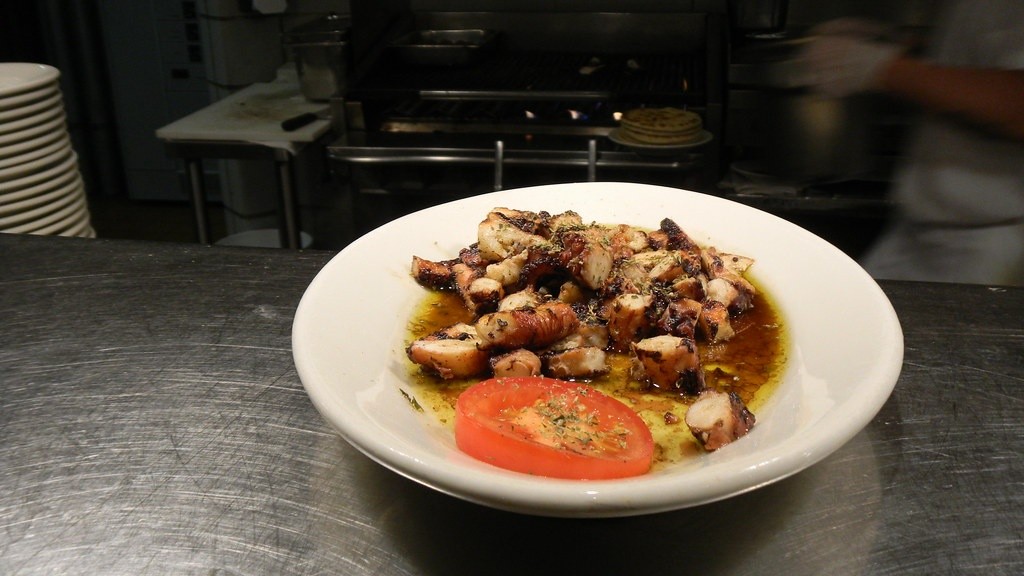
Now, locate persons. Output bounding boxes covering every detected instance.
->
[772,0,1024,288]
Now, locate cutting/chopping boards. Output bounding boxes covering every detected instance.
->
[154,82,360,142]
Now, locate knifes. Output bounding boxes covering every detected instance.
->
[281,102,363,132]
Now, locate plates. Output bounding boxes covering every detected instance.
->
[606,125,713,149]
[1,63,95,238]
[290,183,903,514]
[730,160,818,184]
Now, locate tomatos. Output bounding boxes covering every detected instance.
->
[455,375,654,480]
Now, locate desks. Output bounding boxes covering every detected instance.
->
[166,82,337,250]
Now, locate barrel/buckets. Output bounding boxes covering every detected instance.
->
[213,229,314,249]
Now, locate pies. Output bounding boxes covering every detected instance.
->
[618,108,702,144]
